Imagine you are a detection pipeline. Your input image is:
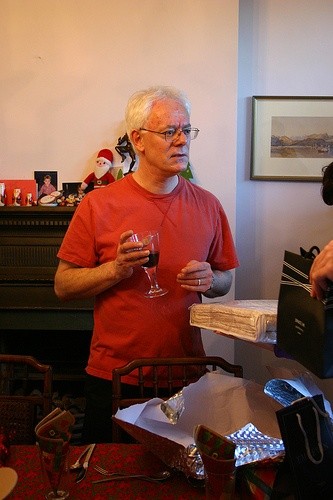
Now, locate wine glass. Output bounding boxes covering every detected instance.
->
[125,231,168,299]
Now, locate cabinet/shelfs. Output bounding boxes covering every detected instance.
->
[0,206,94,395]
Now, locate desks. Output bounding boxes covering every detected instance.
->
[0,443,276,500]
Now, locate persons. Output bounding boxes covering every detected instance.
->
[38,175,56,200]
[309,162,333,300]
[54,84,239,444]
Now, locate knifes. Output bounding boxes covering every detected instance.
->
[76,444,97,484]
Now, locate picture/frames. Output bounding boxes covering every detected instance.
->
[250,96,333,181]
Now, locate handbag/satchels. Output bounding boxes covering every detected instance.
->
[276,394,333,500]
[277,246,333,379]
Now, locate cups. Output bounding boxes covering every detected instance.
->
[205,470,238,500]
[42,451,70,500]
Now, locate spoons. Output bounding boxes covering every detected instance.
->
[92,471,172,483]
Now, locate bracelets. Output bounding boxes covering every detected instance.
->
[209,276,215,291]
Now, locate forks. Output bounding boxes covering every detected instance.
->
[93,466,153,476]
[69,444,92,470]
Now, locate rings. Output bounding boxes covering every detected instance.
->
[198,278,201,286]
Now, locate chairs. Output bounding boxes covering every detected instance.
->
[111,357,244,443]
[1,354,52,445]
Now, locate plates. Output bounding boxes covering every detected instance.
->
[0,467,18,500]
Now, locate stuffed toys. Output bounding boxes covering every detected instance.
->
[81,149,115,191]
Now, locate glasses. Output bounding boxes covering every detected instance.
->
[141,127,199,142]
